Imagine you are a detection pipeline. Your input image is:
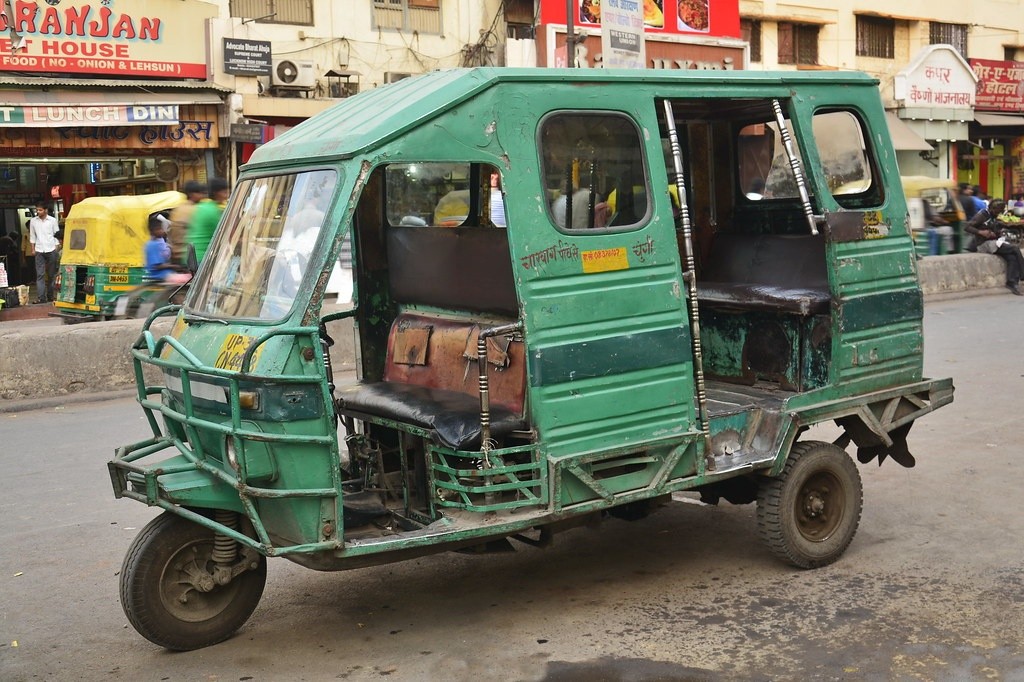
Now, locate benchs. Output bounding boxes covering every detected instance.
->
[321,302,526,448]
[685,233,834,315]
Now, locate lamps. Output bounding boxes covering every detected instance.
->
[920,144,939,168]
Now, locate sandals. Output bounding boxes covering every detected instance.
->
[32,299,46,304]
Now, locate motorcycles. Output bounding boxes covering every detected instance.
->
[106,66,955,653]
[47,191,187,325]
[116,242,206,321]
[834,176,959,257]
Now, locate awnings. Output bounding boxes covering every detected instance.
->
[766,108,935,156]
[0,76,236,128]
[974,111,1024,125]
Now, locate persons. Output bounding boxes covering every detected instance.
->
[288,187,326,277]
[489,169,682,240]
[144,178,229,286]
[922,179,1024,296]
[745,176,765,200]
[0,200,64,304]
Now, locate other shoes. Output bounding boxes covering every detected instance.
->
[1006,283,1023,295]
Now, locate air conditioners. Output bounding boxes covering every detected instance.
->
[270,58,316,87]
